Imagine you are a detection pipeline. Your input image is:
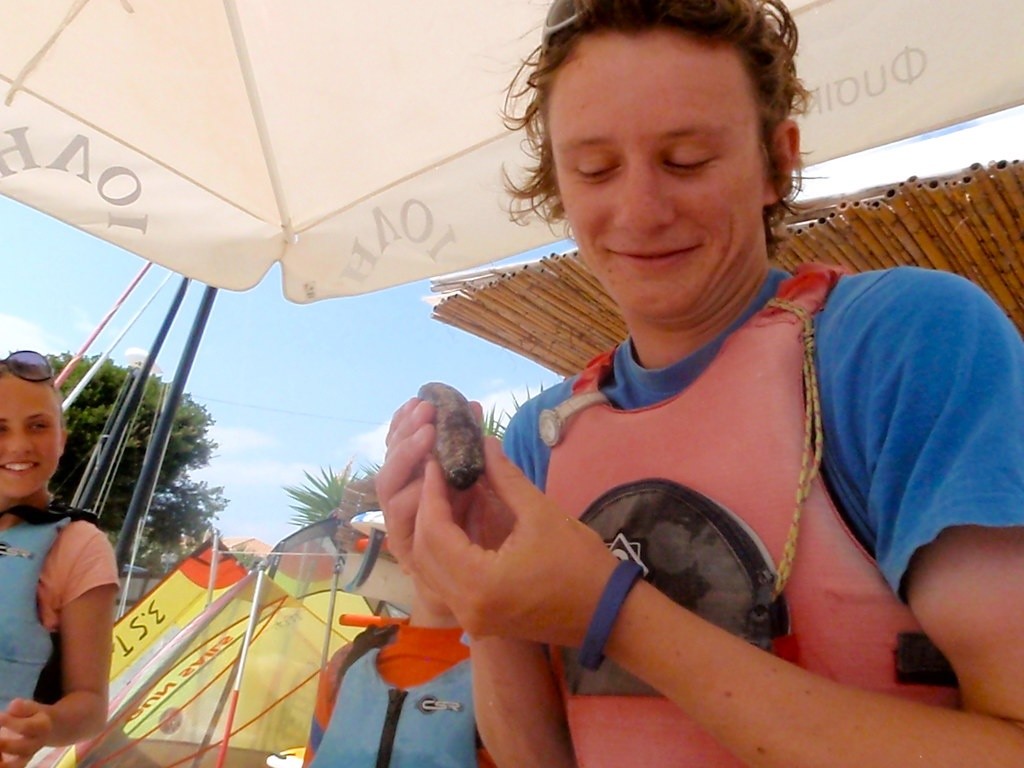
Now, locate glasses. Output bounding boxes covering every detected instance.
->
[1,350,54,382]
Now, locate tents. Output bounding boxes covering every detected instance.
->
[19,511,406,768]
[4,0,1024,302]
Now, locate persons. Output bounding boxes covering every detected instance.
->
[373,0,1024,768]
[304,570,493,766]
[0,359,120,768]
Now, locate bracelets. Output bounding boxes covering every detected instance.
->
[578,551,643,675]
